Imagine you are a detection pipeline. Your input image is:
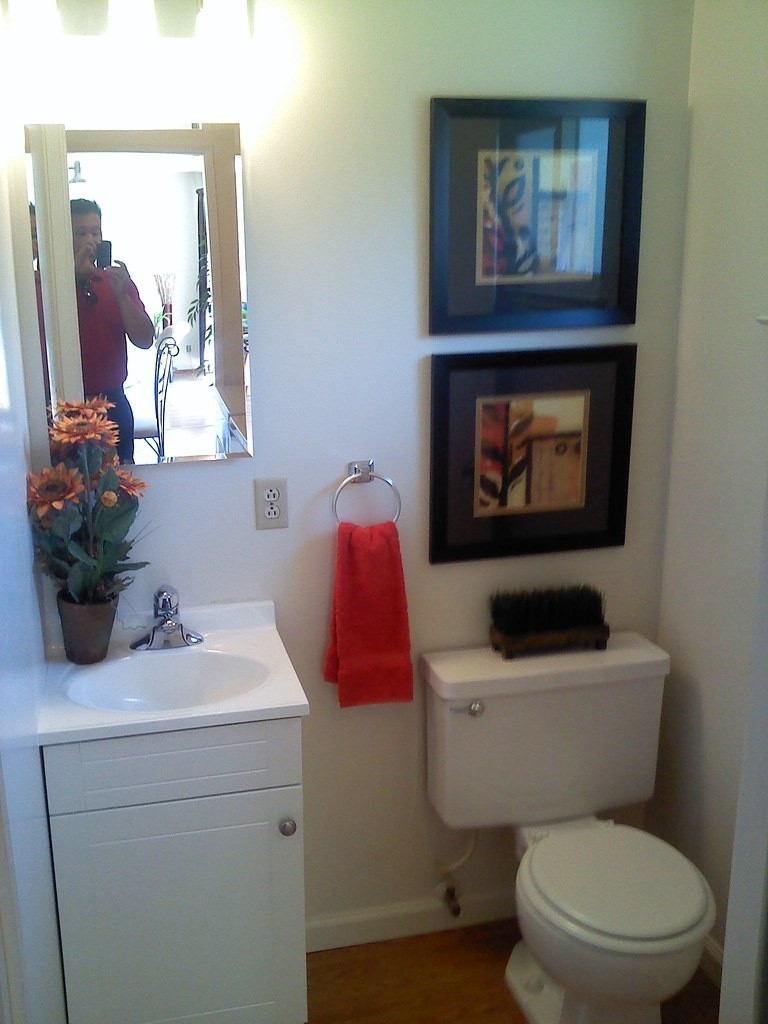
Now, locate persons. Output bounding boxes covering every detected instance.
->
[71,197,156,464]
[28,202,52,425]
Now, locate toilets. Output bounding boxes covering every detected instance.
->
[421,629,718,1023]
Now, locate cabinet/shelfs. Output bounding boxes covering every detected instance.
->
[38,719,310,1024]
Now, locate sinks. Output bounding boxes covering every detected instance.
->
[36,603,313,753]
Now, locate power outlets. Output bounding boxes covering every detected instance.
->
[254,476,290,531]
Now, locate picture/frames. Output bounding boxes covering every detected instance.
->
[428,342,638,565]
[428,98,647,335]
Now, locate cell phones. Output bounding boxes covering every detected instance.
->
[95,241,112,269]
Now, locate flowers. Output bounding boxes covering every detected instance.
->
[24,391,151,604]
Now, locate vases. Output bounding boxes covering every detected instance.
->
[54,591,120,666]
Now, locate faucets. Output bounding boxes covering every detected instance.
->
[130,583,204,655]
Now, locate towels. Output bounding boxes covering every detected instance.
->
[320,517,417,711]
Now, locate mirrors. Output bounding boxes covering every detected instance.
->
[20,128,254,476]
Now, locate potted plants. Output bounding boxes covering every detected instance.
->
[488,583,612,656]
